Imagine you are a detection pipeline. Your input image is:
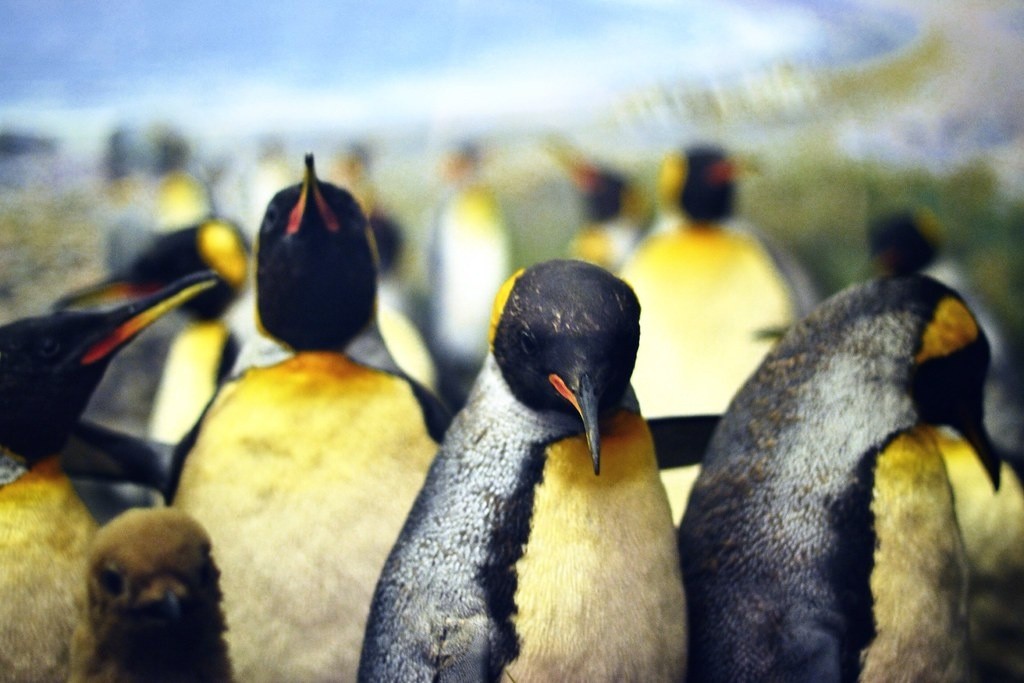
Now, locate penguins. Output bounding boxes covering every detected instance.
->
[0,122,1024,682]
